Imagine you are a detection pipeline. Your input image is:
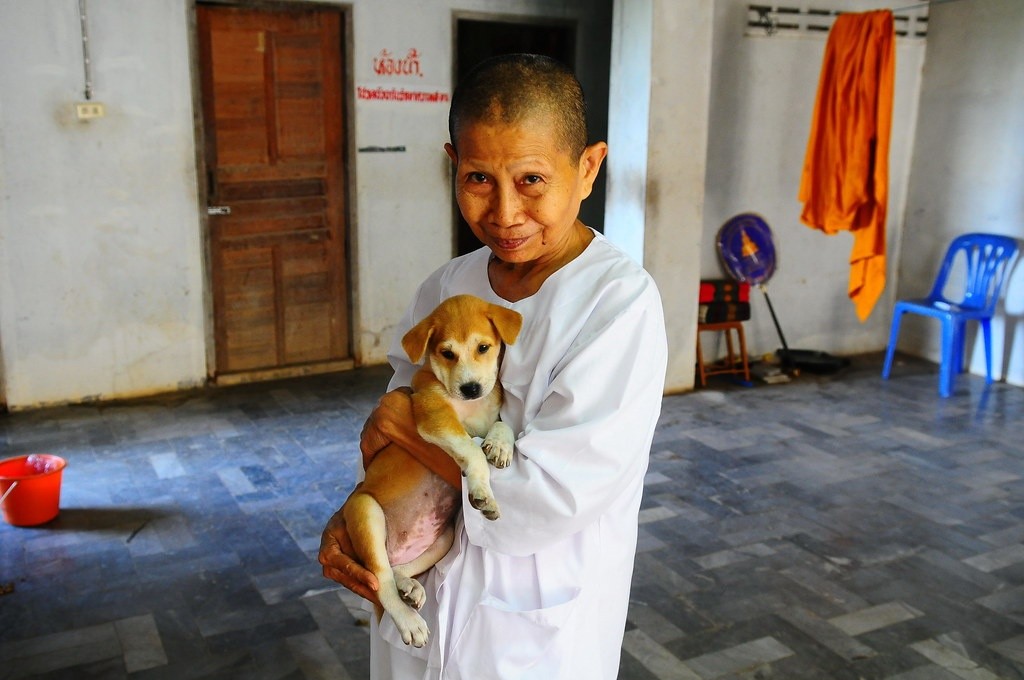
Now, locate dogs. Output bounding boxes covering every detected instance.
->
[341,295,522,649]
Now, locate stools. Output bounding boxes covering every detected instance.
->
[695,319,754,393]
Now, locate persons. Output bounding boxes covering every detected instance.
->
[317,54,669,679]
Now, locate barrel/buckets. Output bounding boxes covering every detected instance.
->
[0,452,67,526]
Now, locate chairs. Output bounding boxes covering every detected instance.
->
[882,231,1019,399]
